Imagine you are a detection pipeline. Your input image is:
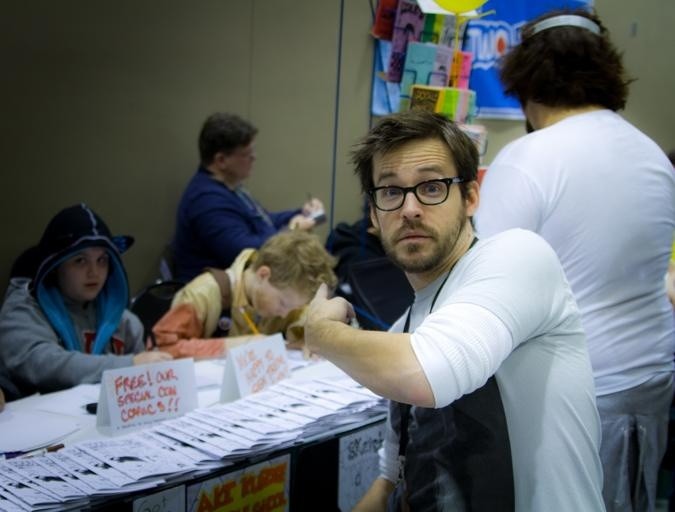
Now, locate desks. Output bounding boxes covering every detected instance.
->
[64,413,413,512]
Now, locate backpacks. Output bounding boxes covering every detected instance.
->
[130,268,233,345]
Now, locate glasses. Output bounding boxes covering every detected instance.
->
[362,174,477,212]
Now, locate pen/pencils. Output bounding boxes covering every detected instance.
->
[239,305,259,336]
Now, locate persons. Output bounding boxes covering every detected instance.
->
[472,7,675,512]
[304,105,608,512]
[664,230,675,308]
[0,203,172,403]
[171,111,327,296]
[153,228,338,359]
